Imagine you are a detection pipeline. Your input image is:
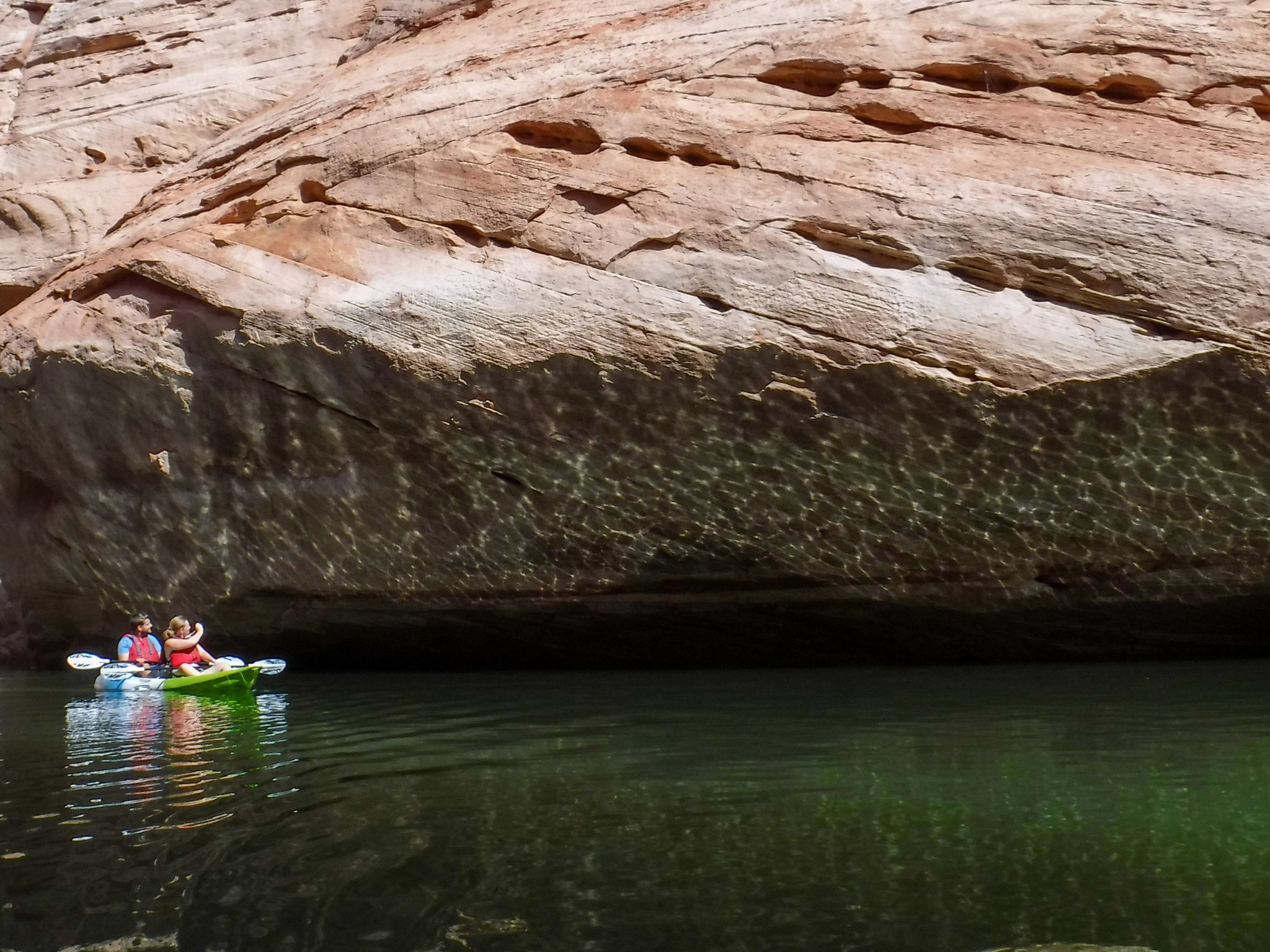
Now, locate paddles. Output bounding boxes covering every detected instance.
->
[100,658,287,682]
[67,652,244,672]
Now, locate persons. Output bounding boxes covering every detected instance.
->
[118,612,180,678]
[163,615,232,676]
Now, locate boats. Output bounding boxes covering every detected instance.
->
[93,663,263,697]
